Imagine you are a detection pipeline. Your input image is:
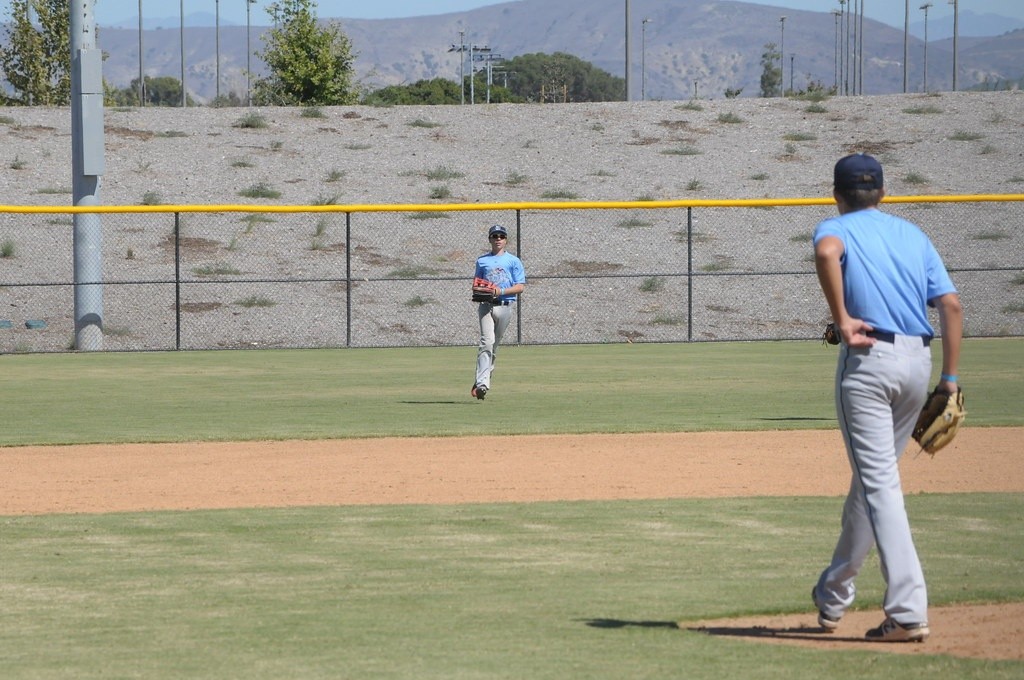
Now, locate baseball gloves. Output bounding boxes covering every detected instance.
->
[471,277,500,303]
[911,384,968,455]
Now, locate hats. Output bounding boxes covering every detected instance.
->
[488,226,507,238]
[833,154,883,190]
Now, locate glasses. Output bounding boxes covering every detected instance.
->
[490,234,507,239]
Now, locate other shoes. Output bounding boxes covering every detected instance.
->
[476,387,486,399]
[472,385,477,397]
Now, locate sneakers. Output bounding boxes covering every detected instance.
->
[865,617,930,642]
[812,585,841,628]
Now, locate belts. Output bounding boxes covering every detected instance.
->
[488,300,509,305]
[866,329,930,347]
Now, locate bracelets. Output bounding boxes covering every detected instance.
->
[938,371,957,383]
[501,288,504,296]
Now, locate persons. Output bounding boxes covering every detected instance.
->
[471,225,526,400]
[812,155,968,642]
